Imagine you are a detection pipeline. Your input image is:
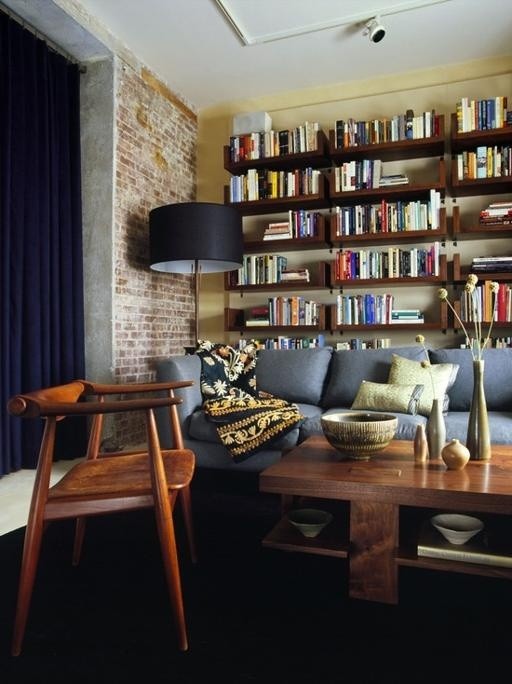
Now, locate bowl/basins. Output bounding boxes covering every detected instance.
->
[321,412,397,460]
[431,513,485,546]
[288,507,333,540]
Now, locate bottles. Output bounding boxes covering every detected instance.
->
[414,424,427,462]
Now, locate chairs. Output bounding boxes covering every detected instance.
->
[9,380,199,658]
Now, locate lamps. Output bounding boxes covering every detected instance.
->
[144,199,243,346]
[362,13,386,45]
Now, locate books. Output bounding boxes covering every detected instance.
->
[229,95,511,349]
[416,534,511,569]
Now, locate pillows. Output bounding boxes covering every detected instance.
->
[255,346,333,405]
[350,379,425,417]
[388,353,461,419]
[429,349,511,414]
[324,345,429,410]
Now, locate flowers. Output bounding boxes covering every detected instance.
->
[415,334,437,401]
[435,271,512,361]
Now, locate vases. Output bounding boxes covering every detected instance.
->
[427,400,446,459]
[466,359,491,459]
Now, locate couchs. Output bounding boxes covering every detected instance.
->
[158,340,512,475]
